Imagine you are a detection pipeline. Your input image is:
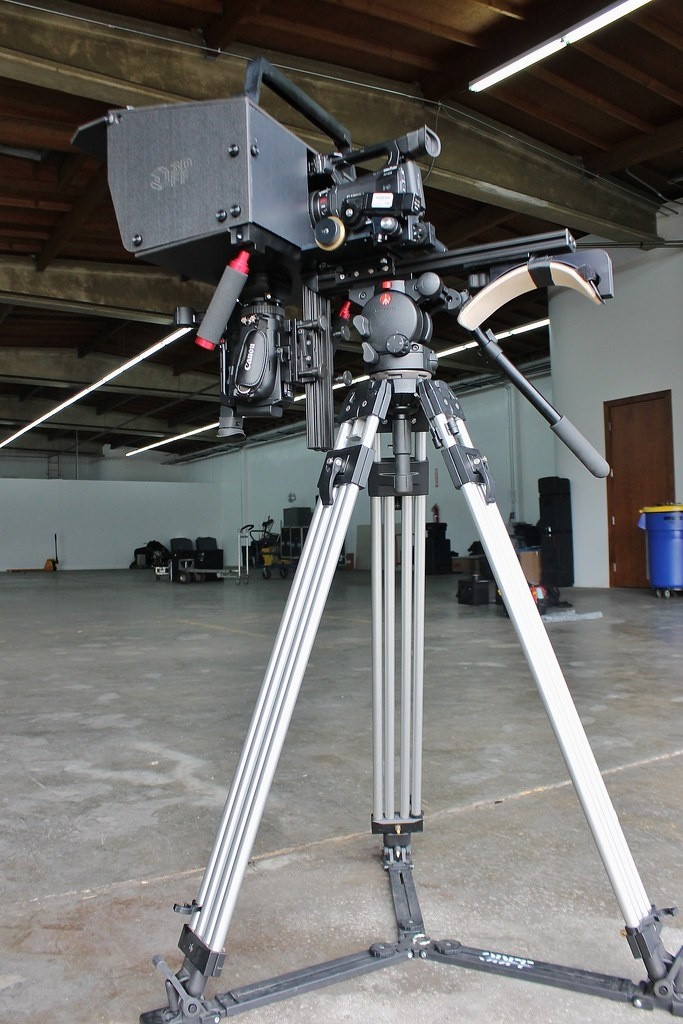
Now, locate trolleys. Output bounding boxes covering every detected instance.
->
[177,523,254,585]
[249,529,294,580]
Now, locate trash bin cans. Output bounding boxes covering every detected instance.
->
[638,501,683,597]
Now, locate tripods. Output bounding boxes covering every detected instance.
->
[142,248,683,1024]
